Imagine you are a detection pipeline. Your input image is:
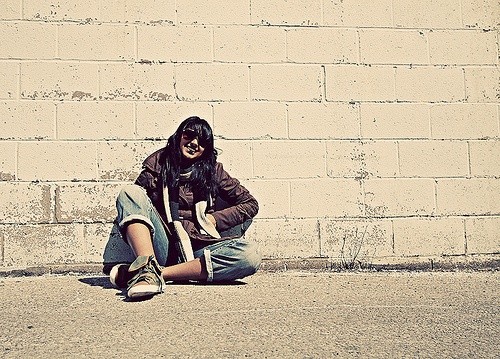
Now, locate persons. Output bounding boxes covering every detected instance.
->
[109,116,261,297]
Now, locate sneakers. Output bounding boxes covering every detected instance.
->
[109,255,167,297]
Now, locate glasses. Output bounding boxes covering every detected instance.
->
[181,127,207,147]
